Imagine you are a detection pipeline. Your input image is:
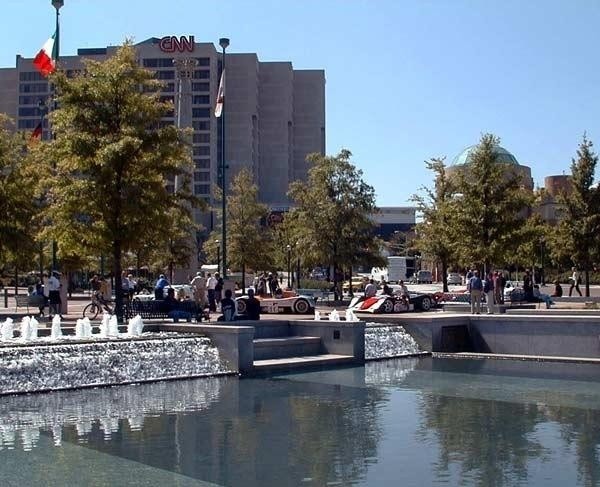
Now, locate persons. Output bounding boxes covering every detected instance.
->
[191,272,281,321]
[551,279,562,297]
[331,275,411,307]
[121,270,129,300]
[27,270,65,322]
[128,274,138,299]
[89,275,103,314]
[466,266,555,315]
[92,275,111,313]
[177,289,210,322]
[568,266,582,296]
[164,289,192,322]
[155,273,171,299]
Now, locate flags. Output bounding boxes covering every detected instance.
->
[213,68,225,117]
[33,19,59,77]
[26,122,41,149]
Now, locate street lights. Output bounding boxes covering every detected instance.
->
[284,243,294,287]
[213,238,222,276]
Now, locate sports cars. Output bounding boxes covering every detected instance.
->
[345,293,433,317]
[233,292,319,315]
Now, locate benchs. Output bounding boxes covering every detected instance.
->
[14,295,50,314]
[437,292,485,313]
[509,288,546,308]
[126,299,210,323]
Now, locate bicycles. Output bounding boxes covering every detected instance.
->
[134,296,155,318]
[82,291,132,319]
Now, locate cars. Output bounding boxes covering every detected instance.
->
[164,275,219,303]
[16,270,64,287]
[310,266,342,281]
[342,275,366,292]
[367,256,541,301]
